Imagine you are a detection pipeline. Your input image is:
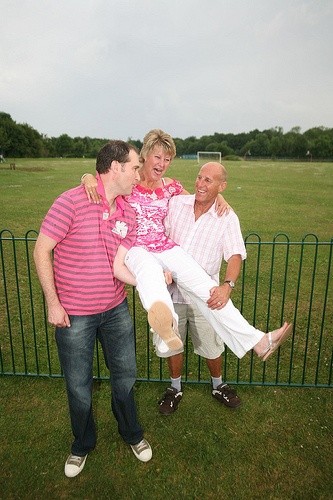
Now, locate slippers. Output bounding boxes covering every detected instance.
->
[259,324,292,361]
[147,301,183,351]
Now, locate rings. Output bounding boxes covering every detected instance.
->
[90,191,92,193]
[218,302,222,306]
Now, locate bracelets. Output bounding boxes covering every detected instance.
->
[81,173,88,182]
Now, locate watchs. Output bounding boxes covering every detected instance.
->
[224,280,235,289]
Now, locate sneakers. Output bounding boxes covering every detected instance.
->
[158,387,183,414]
[65,453,88,477]
[130,439,152,462]
[211,385,241,407]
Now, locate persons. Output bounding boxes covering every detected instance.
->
[82,130,293,362]
[33,140,172,478]
[158,161,247,416]
